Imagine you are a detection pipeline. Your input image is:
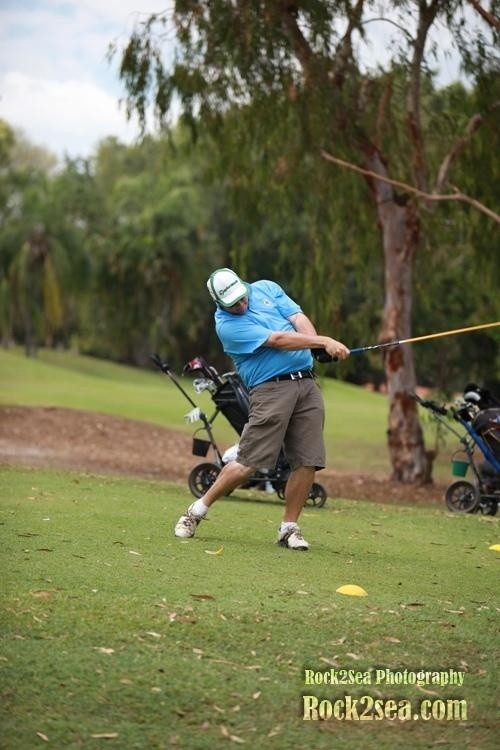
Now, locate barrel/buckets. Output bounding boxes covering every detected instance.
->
[451,449,471,477]
[192,428,211,457]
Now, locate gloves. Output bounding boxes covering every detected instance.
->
[186,408,200,424]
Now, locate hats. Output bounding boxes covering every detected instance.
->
[207,268,250,307]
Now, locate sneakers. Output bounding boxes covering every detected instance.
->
[277,524,309,551]
[174,502,207,538]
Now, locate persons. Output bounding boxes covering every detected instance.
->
[174,267,351,550]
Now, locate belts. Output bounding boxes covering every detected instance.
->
[264,371,315,382]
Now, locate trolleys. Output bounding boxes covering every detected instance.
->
[150,353,328,509]
[411,389,500,515]
